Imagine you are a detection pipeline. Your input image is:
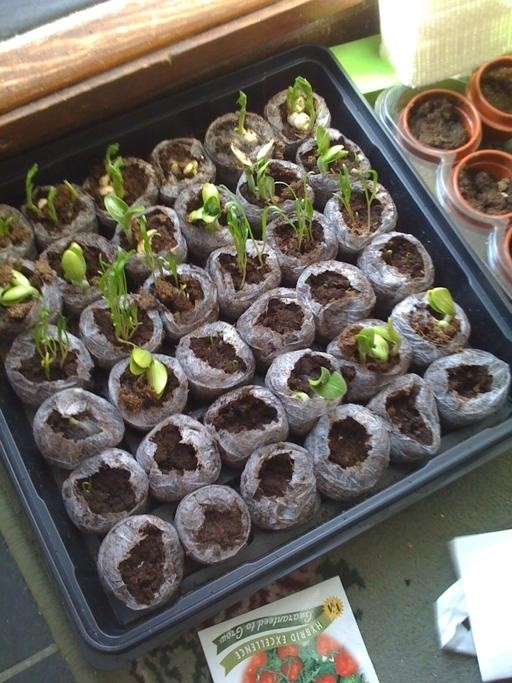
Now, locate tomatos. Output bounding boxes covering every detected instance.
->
[241,634,358,683]
